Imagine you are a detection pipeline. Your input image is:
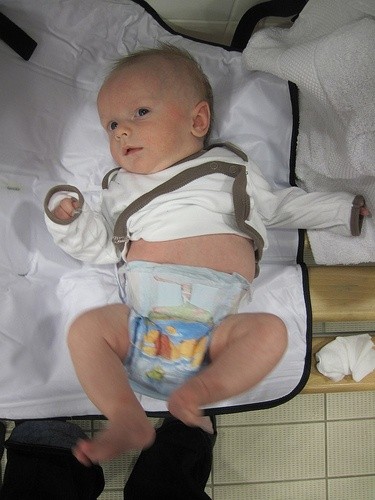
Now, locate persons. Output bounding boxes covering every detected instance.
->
[43,41,369,467]
